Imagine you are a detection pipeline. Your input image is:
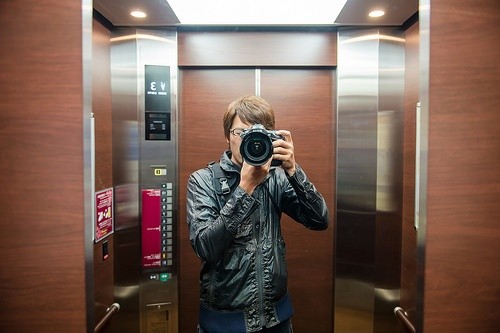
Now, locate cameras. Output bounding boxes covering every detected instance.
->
[239,124,285,168]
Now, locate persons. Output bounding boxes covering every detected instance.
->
[184,94,331,333]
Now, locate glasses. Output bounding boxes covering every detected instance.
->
[229,128,245,138]
